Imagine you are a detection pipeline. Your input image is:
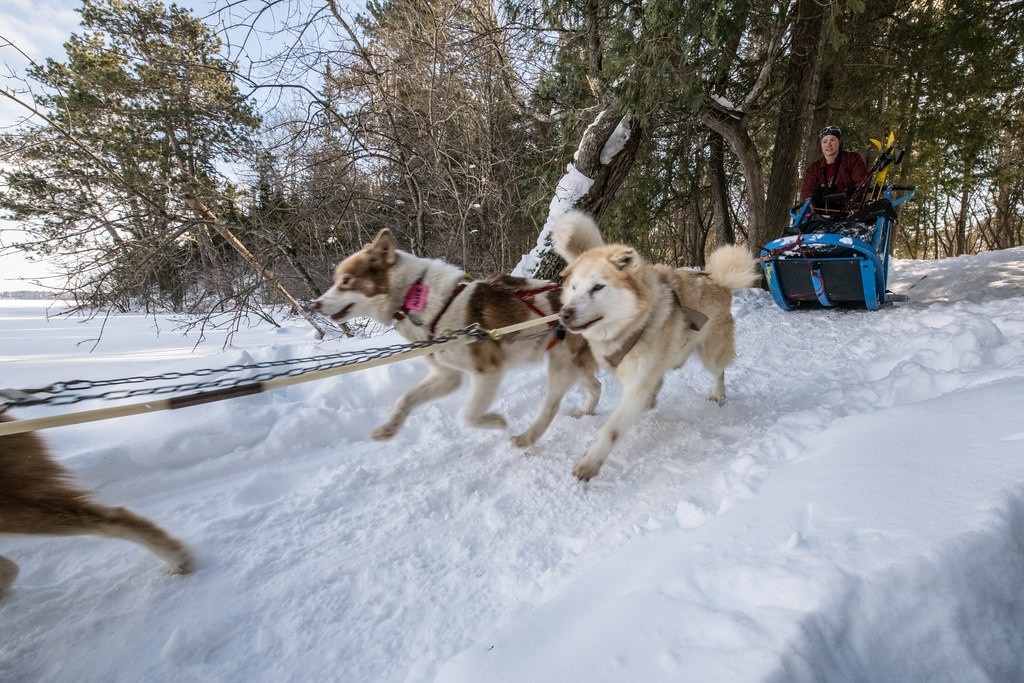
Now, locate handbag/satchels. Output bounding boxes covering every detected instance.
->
[858,197,899,226]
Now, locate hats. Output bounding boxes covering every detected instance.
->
[820,125,841,142]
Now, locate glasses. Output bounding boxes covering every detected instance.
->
[820,125,841,140]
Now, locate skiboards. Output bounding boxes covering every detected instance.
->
[860,131,895,203]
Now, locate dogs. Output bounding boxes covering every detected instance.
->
[557,243,764,483]
[0,413,196,600]
[311,210,604,448]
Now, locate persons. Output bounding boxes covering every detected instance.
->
[799,125,870,221]
